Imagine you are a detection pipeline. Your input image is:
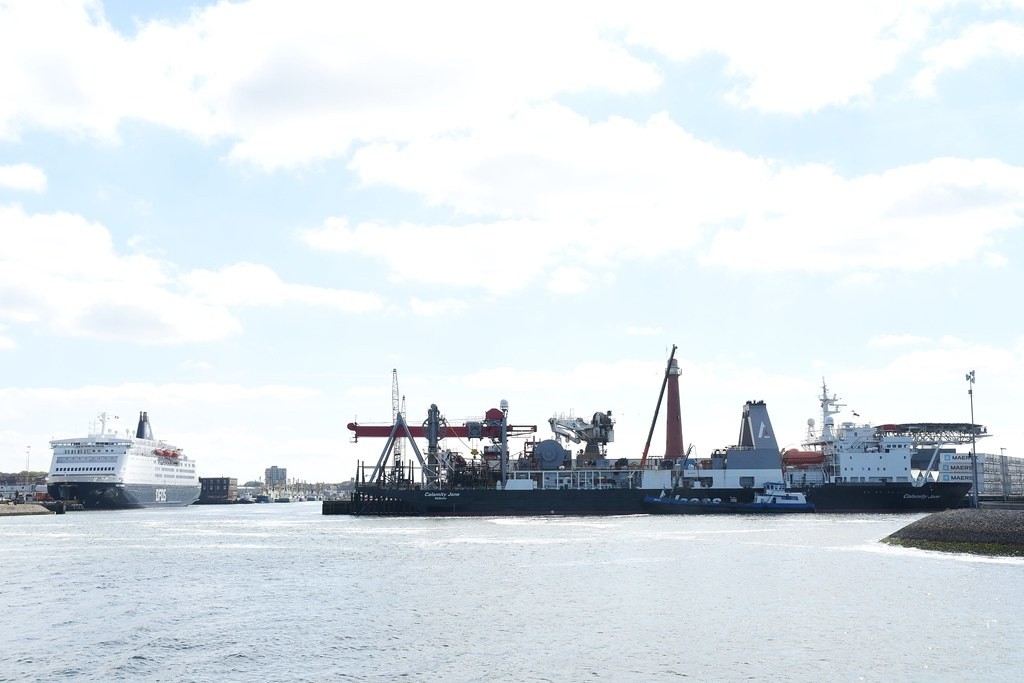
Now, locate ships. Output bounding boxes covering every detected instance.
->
[321,343,990,513]
[46,412,202,511]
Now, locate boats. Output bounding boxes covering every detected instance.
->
[642,442,816,513]
[234,477,354,502]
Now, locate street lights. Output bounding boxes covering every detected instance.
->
[964,369,980,509]
